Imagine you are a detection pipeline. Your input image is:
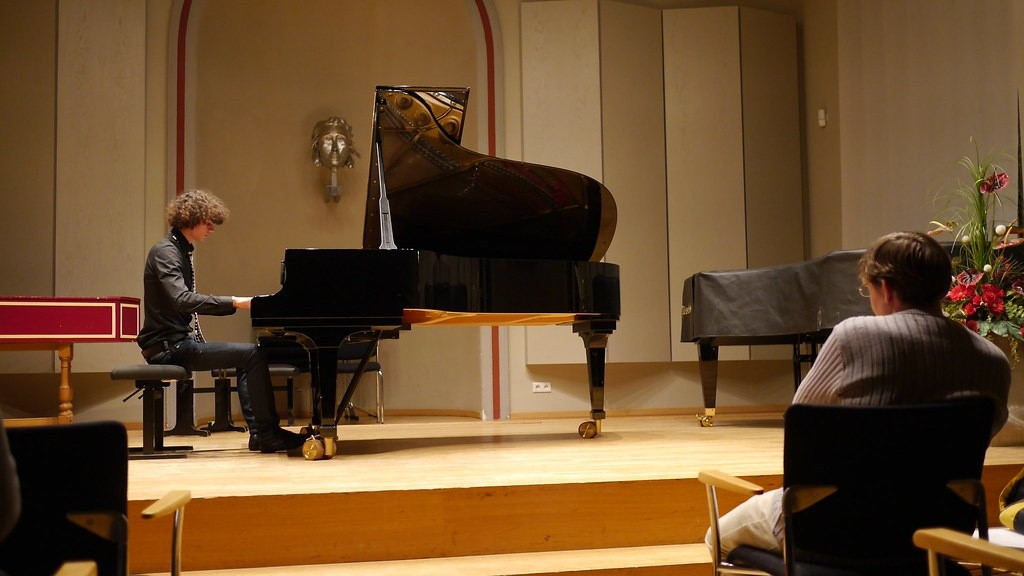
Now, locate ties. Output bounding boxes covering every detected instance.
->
[188,251,207,343]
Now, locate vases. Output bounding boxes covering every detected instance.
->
[984,333,1024,447]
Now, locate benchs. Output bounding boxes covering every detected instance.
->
[193,366,299,431]
[110,364,192,460]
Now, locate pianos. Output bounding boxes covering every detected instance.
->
[681,240,997,428]
[0,294,143,422]
[246,83,622,460]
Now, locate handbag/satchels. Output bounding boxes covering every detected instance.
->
[999,467,1023,535]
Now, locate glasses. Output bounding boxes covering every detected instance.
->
[858,281,870,298]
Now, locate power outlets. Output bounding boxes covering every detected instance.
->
[532,382,551,392]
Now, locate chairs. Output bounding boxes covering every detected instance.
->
[336,340,385,424]
[699,402,1024,576]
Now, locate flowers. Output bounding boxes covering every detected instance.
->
[925,136,1024,372]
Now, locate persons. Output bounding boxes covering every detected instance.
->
[136,189,309,454]
[310,115,353,168]
[702,232,1011,568]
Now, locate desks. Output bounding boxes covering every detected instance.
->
[0,296,141,426]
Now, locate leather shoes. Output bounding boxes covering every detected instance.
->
[249,431,260,451]
[258,425,310,453]
